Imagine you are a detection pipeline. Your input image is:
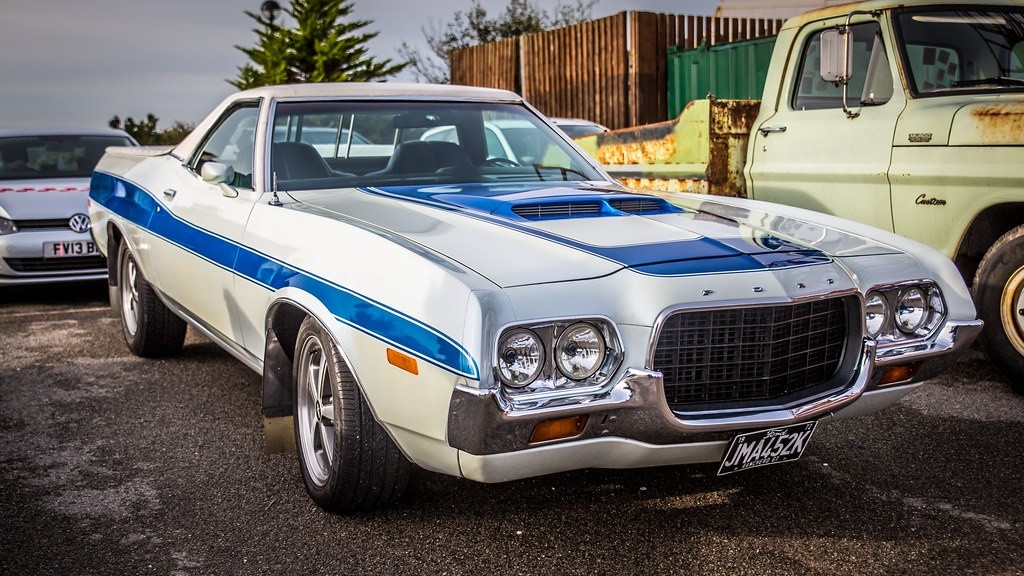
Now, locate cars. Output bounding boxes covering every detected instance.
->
[81,79,986,519]
[0,126,142,286]
[418,116,614,172]
[219,123,374,149]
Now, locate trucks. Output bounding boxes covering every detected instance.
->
[540,0,1024,400]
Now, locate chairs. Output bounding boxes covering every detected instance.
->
[362,138,473,178]
[271,140,356,182]
